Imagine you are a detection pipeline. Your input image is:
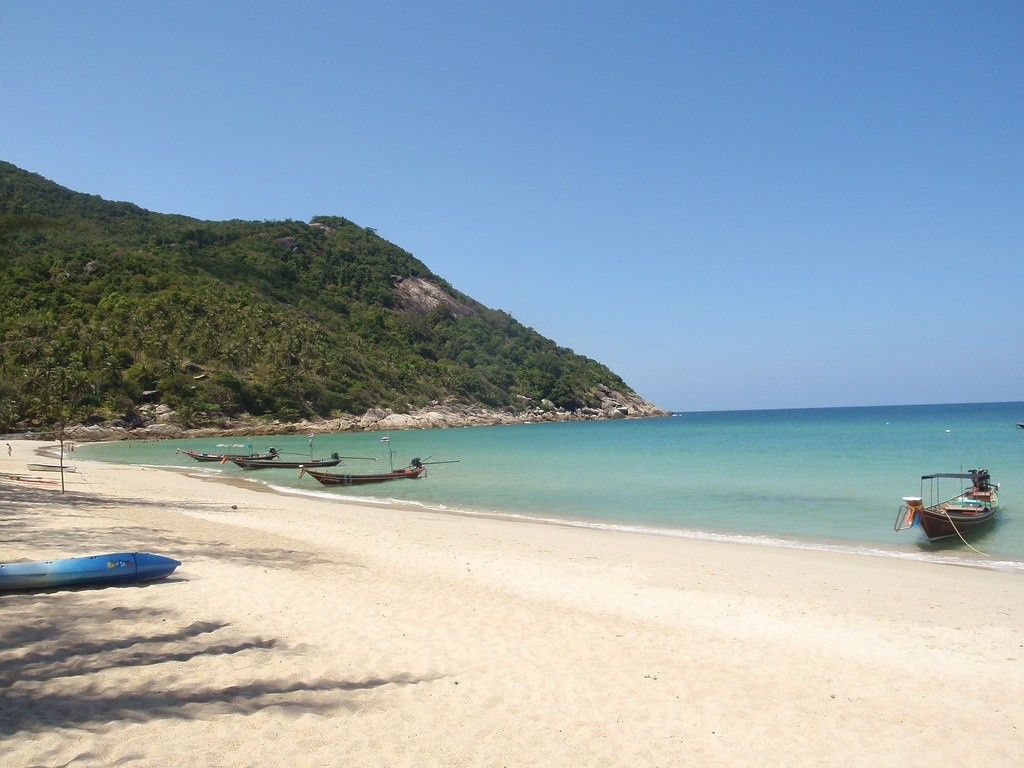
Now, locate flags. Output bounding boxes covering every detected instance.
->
[379,435,390,445]
[248,436,255,448]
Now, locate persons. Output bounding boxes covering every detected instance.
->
[68,443,71,452]
[71,443,75,452]
[6,443,13,457]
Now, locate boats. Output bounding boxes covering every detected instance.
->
[1016,423,1024,430]
[174,443,285,467]
[894,466,1000,543]
[299,430,428,492]
[26,462,79,474]
[0,552,182,593]
[221,431,342,476]
